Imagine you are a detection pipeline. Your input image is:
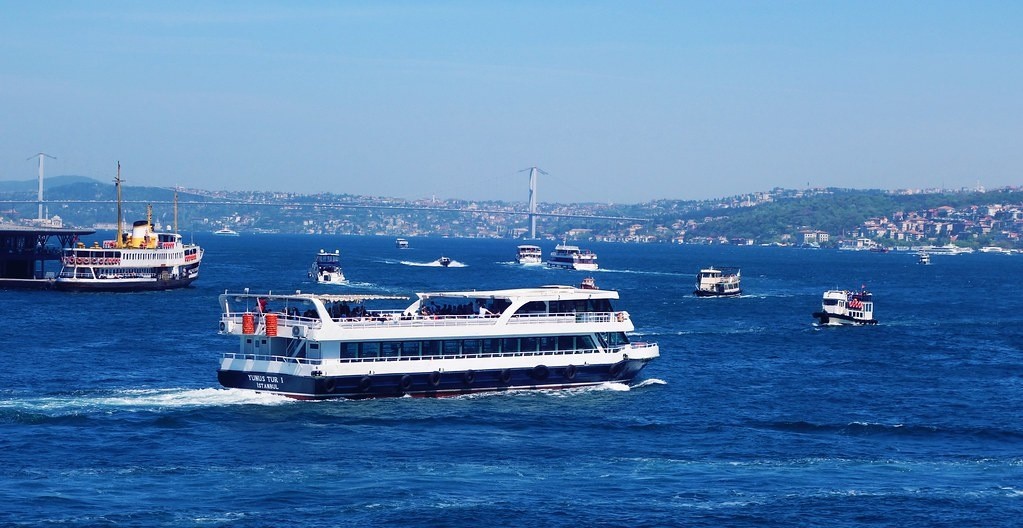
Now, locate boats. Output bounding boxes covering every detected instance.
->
[395,238,409,248]
[547,241,599,271]
[515,245,542,264]
[215,228,239,237]
[55,160,205,291]
[693,266,742,297]
[812,290,878,325]
[870,247,890,253]
[216,278,661,400]
[308,249,346,282]
[919,253,930,265]
[439,257,451,267]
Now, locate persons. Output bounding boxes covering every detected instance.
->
[479,304,492,318]
[301,302,380,322]
[292,307,300,320]
[422,301,439,320]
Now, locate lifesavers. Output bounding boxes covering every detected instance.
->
[564,364,576,380]
[360,377,373,392]
[849,301,862,308]
[292,326,300,335]
[532,365,549,380]
[220,322,225,331]
[68,257,121,264]
[400,375,413,390]
[163,242,174,247]
[429,371,443,388]
[464,369,476,384]
[617,312,624,322]
[323,377,336,392]
[500,369,513,383]
